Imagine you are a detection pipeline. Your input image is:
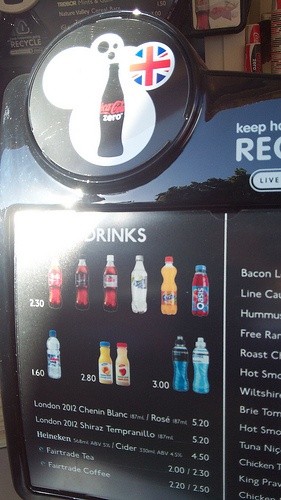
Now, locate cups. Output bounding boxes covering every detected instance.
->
[195,1,210,29]
[243,0,281,74]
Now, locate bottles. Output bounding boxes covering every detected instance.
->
[97,63,124,157]
[47,253,212,395]
